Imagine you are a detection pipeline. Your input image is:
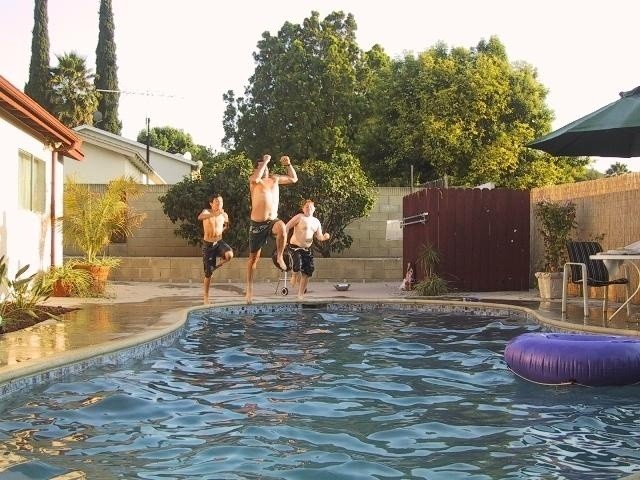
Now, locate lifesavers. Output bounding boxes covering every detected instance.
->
[504,334,639,387]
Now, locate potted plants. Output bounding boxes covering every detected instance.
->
[32,172,148,301]
[530,198,581,300]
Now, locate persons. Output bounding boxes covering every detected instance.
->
[284,199,331,300]
[244,153,299,306]
[197,191,234,308]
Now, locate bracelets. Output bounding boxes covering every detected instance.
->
[283,164,292,167]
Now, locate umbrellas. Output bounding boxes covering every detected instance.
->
[521,84,640,162]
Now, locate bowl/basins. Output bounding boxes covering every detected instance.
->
[332,282,352,290]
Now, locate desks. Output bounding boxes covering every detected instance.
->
[590,254,640,322]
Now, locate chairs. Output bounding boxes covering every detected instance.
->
[561,241,634,318]
